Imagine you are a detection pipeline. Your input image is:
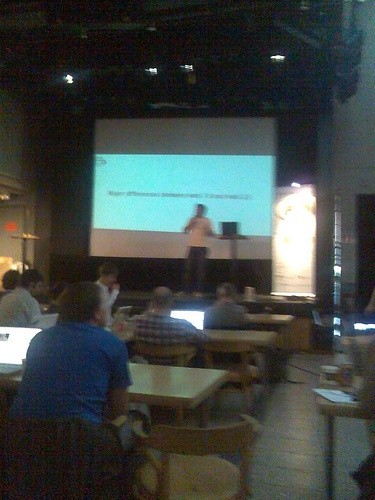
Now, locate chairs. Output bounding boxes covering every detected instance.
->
[136,413,259,500]
[201,341,266,418]
[133,338,193,369]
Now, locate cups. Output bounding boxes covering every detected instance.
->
[244,286,256,301]
[319,366,341,387]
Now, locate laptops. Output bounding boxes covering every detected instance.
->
[170,309,206,334]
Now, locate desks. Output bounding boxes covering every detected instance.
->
[1,295,301,500]
[314,364,375,500]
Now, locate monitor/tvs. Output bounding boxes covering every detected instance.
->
[222,221,237,236]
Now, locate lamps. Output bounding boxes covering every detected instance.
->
[145,55,157,73]
[269,36,286,63]
[64,66,74,84]
[180,50,194,71]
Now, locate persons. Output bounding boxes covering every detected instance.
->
[95,261,120,326]
[134,288,207,367]
[0,269,44,328]
[175,204,215,297]
[9,281,133,453]
[203,282,249,363]
